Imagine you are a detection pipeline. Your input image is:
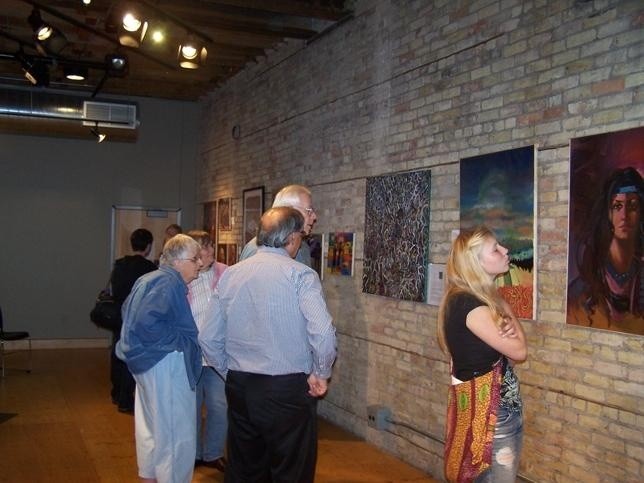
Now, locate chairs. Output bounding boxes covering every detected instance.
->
[0,307,32,377]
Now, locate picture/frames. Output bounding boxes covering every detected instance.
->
[243,185,265,251]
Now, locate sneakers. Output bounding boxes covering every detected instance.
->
[111,398,134,414]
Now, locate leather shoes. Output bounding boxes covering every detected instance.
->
[193,457,228,472]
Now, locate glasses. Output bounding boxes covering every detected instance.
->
[178,256,201,262]
[295,205,312,216]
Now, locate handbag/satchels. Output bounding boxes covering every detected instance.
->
[443,358,505,483]
[89,279,120,330]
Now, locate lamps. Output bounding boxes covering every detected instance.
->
[15,0,209,94]
[89,121,107,143]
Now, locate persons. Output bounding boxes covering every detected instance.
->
[436,226,528,483]
[568,166,644,334]
